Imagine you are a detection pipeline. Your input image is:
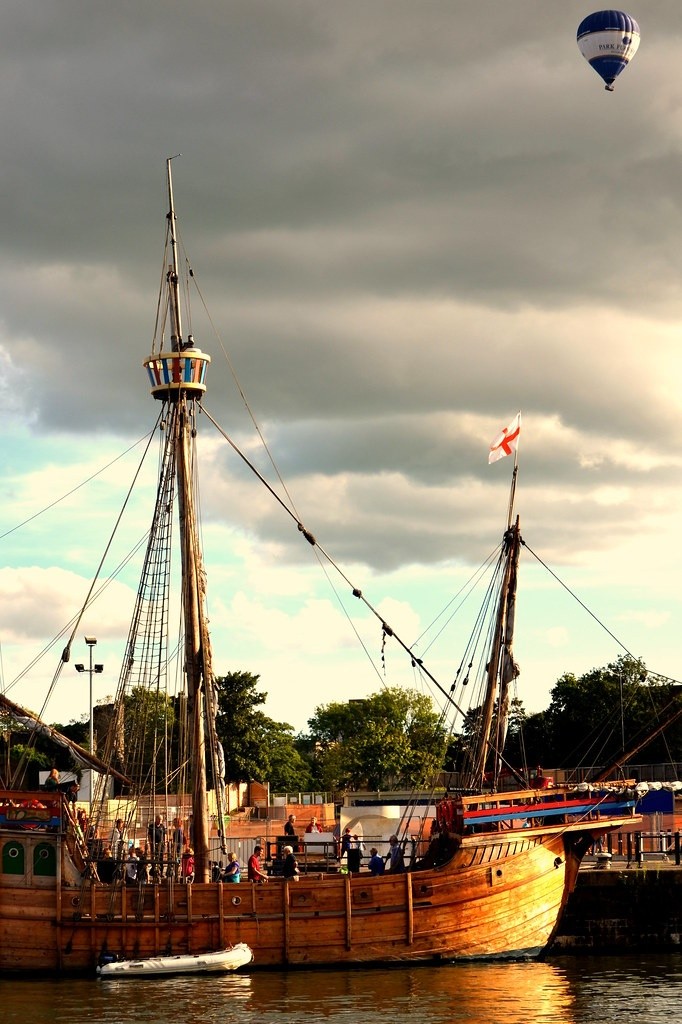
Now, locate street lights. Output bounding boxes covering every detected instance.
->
[75,637,105,821]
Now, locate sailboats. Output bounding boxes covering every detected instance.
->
[1,151,647,968]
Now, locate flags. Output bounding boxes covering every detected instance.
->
[488,411,521,464]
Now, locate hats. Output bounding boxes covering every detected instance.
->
[186,848,194,855]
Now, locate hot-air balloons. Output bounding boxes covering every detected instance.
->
[577,8,642,91]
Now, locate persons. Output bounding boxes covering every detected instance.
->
[0,767,543,882]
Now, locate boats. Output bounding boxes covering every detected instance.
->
[97,942,255,982]
